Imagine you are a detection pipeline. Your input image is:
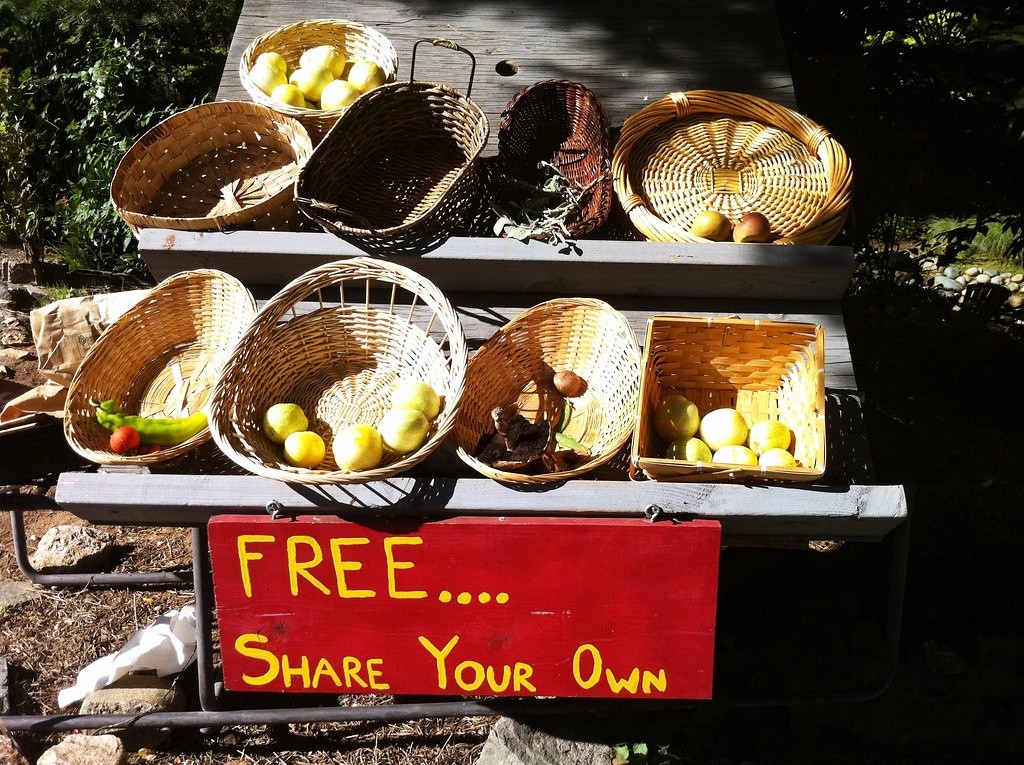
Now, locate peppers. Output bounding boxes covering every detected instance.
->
[88,393,209,447]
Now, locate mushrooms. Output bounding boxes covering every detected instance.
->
[475,406,552,470]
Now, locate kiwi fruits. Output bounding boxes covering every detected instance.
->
[554,371,581,396]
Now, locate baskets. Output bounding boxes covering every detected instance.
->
[207,255,468,486]
[63,269,260,466]
[610,88,855,247]
[491,78,613,240]
[627,313,828,482]
[238,17,399,150]
[291,36,491,239]
[448,298,644,486]
[108,100,315,231]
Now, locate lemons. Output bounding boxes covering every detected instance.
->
[249,45,386,111]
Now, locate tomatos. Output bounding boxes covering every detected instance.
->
[109,426,141,454]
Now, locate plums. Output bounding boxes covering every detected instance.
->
[691,211,772,242]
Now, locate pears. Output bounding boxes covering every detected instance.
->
[262,382,441,472]
[653,395,797,471]
